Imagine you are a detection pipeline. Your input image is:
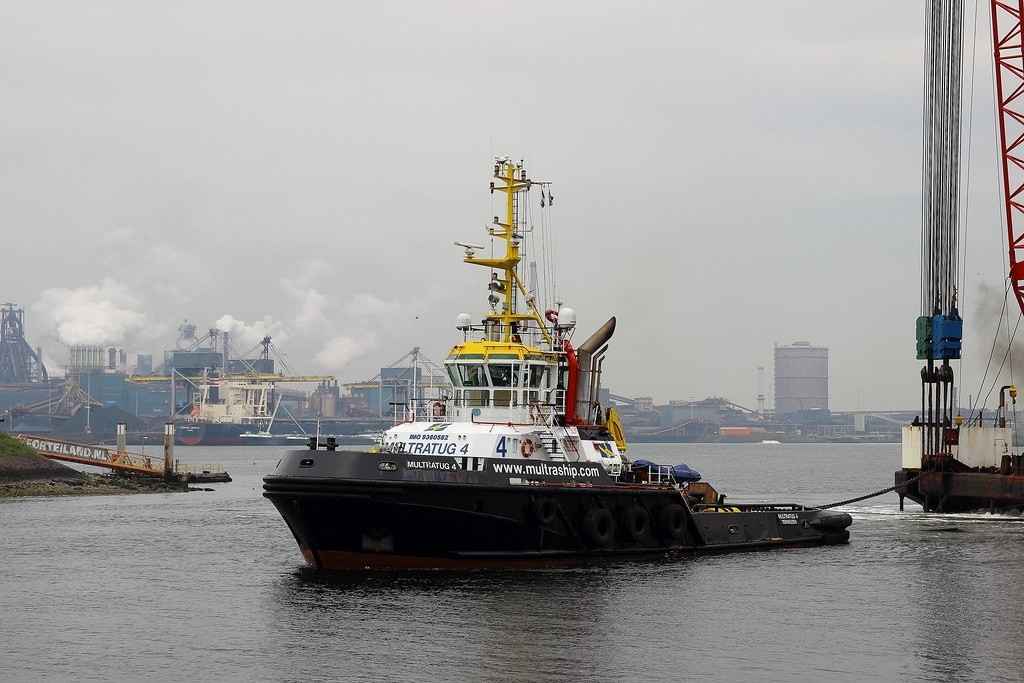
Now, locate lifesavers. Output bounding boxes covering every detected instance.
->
[546,310,558,322]
[622,505,651,541]
[521,439,534,458]
[664,504,689,539]
[584,508,616,546]
[433,402,441,415]
[539,499,557,523]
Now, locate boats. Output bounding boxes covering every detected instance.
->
[263,154,854,569]
[892,0,1023,516]
[173,376,381,446]
